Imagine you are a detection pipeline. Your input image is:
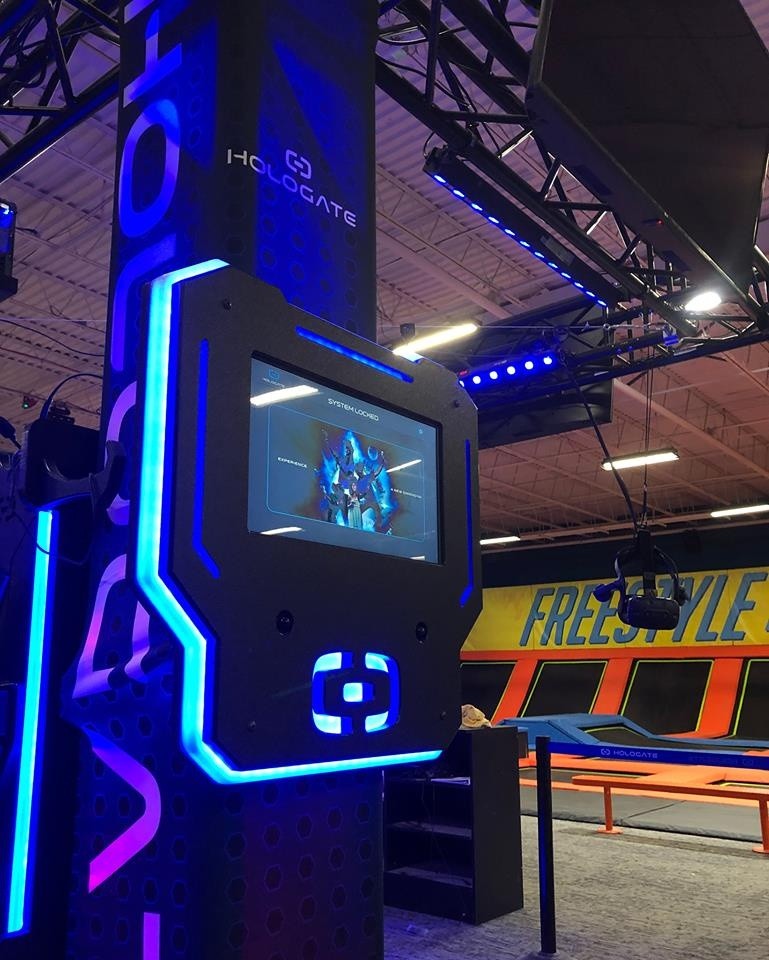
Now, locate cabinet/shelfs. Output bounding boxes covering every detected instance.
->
[384,727,524,925]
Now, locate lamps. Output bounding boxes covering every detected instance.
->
[423,132,623,309]
[599,447,681,472]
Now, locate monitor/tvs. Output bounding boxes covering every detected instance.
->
[245,348,449,570]
[446,302,619,450]
[522,0,769,294]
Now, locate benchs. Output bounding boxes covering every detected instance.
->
[571,775,769,853]
